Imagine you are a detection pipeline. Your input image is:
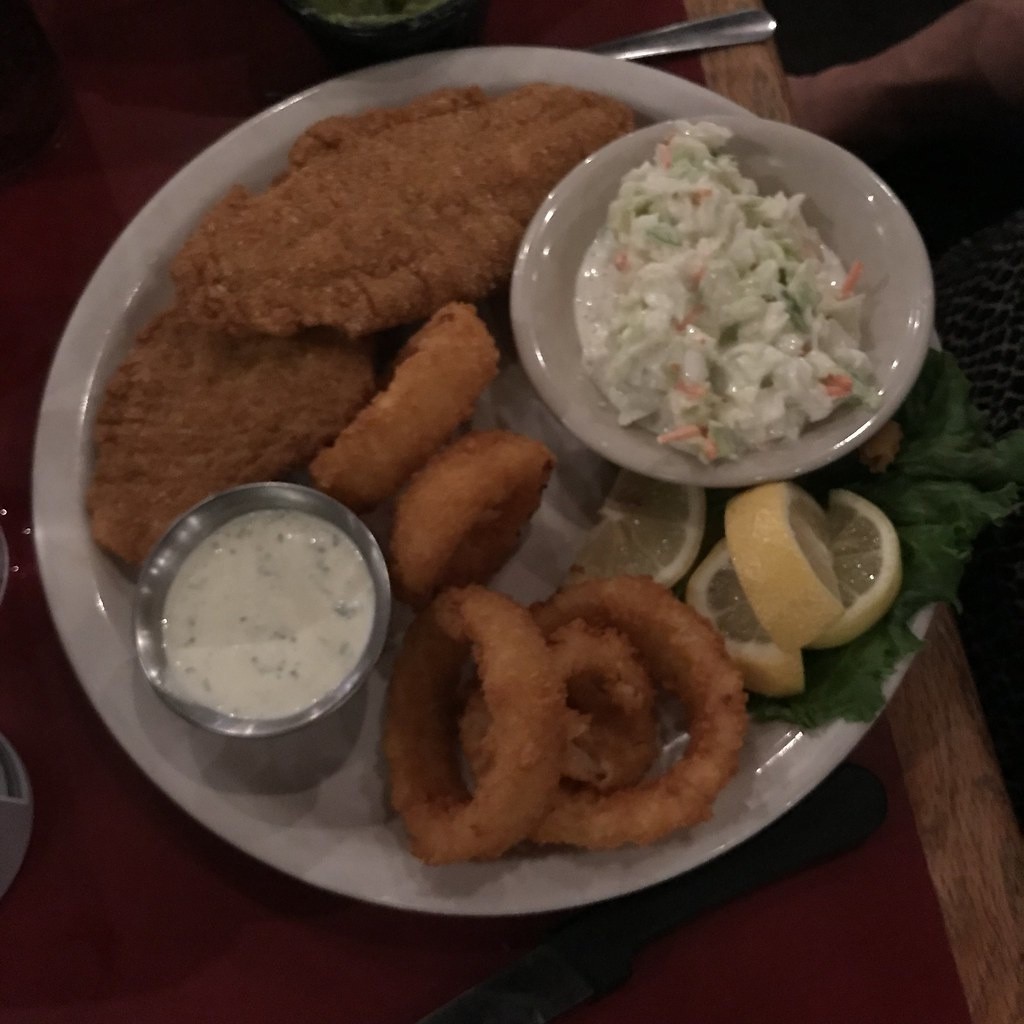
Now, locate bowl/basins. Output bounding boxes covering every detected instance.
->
[510,110,941,490]
[122,481,394,738]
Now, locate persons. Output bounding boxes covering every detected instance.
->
[785,0,1024,152]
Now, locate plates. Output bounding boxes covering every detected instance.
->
[29,44,965,920]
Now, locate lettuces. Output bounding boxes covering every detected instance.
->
[675,347,1024,730]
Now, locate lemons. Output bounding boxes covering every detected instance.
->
[567,465,902,700]
